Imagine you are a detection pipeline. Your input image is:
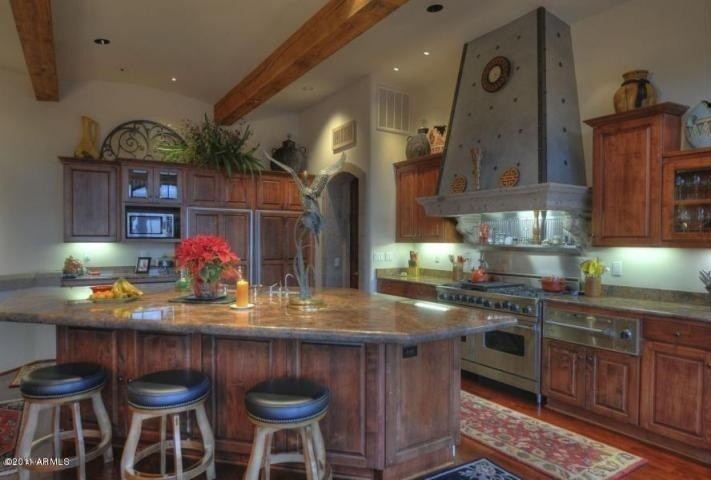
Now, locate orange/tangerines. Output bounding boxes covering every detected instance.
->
[93,290,114,299]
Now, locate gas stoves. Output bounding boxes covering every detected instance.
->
[434,276,576,319]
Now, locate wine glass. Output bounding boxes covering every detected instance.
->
[674,172,710,231]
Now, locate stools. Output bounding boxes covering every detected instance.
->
[15,363,115,479]
[120,370,217,479]
[242,378,333,479]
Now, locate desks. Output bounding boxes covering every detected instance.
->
[0,286,521,480]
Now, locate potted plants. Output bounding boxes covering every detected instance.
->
[538,276,569,292]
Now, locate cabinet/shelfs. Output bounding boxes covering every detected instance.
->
[188,162,254,286]
[582,102,711,249]
[255,170,316,287]
[541,339,641,442]
[114,158,188,243]
[377,278,437,302]
[57,155,120,243]
[392,152,464,243]
[640,315,711,466]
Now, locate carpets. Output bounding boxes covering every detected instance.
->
[417,388,648,480]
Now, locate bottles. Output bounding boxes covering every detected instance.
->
[404,134,430,160]
[612,69,656,112]
[268,139,307,172]
[73,115,102,158]
[471,266,483,282]
[174,271,192,292]
[474,211,567,247]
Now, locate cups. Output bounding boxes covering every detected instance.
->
[408,259,420,279]
[452,262,463,280]
[585,275,602,297]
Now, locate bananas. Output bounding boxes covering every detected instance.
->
[112,276,144,299]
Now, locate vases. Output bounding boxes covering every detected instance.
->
[409,260,420,277]
[192,283,227,298]
[453,267,463,281]
[584,279,602,297]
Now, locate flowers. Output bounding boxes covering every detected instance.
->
[175,235,242,283]
[157,110,266,183]
[579,257,605,278]
[410,251,420,260]
[448,254,469,267]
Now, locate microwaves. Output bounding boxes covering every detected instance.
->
[127,212,174,238]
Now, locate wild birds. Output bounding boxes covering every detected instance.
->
[263,149,347,248]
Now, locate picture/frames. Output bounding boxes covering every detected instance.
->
[135,257,152,274]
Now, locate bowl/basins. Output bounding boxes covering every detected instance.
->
[89,285,113,293]
[538,279,566,292]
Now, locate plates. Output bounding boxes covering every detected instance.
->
[229,303,255,309]
[88,293,143,302]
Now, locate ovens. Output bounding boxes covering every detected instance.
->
[438,302,542,396]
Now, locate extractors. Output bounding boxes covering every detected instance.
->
[414,4,593,219]
